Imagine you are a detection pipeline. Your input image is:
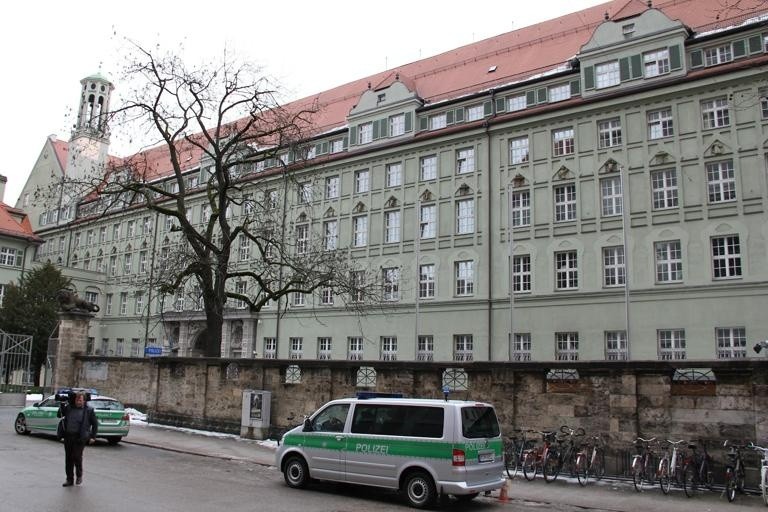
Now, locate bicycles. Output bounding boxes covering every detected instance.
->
[627,435,768,508]
[503,424,607,487]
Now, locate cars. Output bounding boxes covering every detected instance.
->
[12,387,132,447]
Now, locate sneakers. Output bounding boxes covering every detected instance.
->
[63,475,83,486]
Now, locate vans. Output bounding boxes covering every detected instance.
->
[273,390,509,509]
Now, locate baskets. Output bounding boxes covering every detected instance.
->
[542,429,556,443]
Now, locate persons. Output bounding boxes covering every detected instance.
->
[57,391,98,486]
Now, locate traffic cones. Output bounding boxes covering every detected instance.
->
[497,478,512,502]
